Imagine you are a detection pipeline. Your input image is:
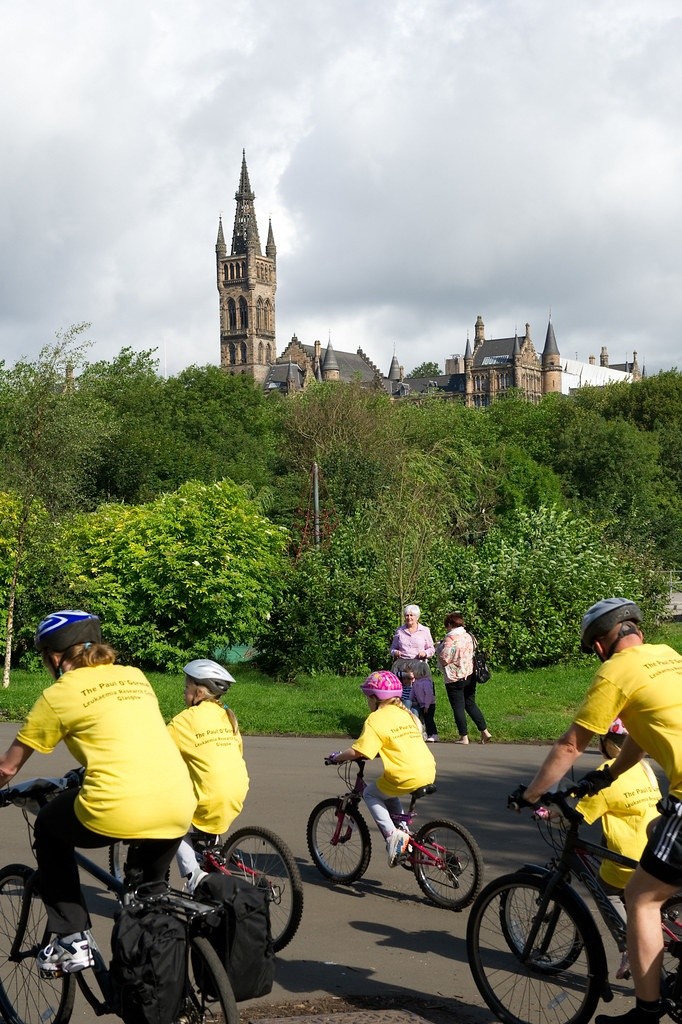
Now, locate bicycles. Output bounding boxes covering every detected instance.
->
[0,766,241,1024]
[306,752,486,911]
[109,824,305,954]
[465,783,682,1024]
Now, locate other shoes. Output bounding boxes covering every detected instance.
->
[422,732,428,742]
[478,732,492,744]
[456,736,470,744]
[594,1007,660,1024]
[426,734,440,741]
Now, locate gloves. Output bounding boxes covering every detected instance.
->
[507,783,535,811]
[328,752,345,766]
[577,763,613,799]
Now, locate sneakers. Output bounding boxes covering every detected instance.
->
[36,931,95,972]
[386,829,409,867]
[181,866,208,899]
[615,949,632,979]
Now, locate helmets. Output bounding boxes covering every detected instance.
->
[360,670,403,700]
[581,596,643,654]
[34,610,102,652]
[600,717,630,735]
[183,659,237,684]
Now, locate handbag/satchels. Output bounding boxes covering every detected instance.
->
[109,903,189,1024]
[189,871,273,1002]
[467,632,491,683]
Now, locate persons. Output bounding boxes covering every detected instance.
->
[329,671,437,868]
[507,598,682,1024]
[546,718,663,979]
[389,604,493,745]
[0,609,199,974]
[165,659,250,895]
